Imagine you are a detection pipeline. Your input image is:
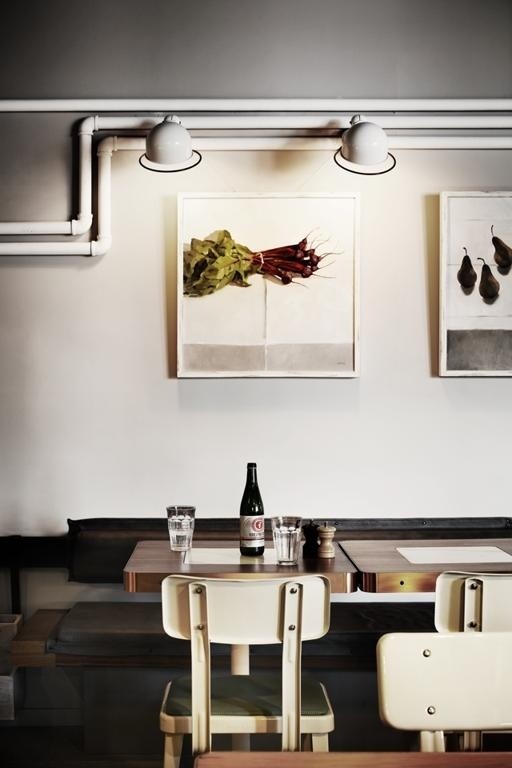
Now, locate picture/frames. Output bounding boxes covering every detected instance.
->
[438,192,512,378]
[176,191,362,379]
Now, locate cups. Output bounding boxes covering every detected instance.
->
[270,514,303,566]
[166,506,196,552]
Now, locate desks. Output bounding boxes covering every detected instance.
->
[193,751,511,768]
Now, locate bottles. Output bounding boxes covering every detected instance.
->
[240,463,265,556]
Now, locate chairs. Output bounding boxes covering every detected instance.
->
[157,574,340,768]
[376,633,511,753]
[378,570,512,753]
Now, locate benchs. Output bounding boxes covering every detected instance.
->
[11,515,512,671]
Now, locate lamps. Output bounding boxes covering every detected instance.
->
[138,115,200,174]
[334,116,399,178]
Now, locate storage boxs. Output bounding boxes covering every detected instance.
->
[0,670,26,720]
[0,613,23,674]
